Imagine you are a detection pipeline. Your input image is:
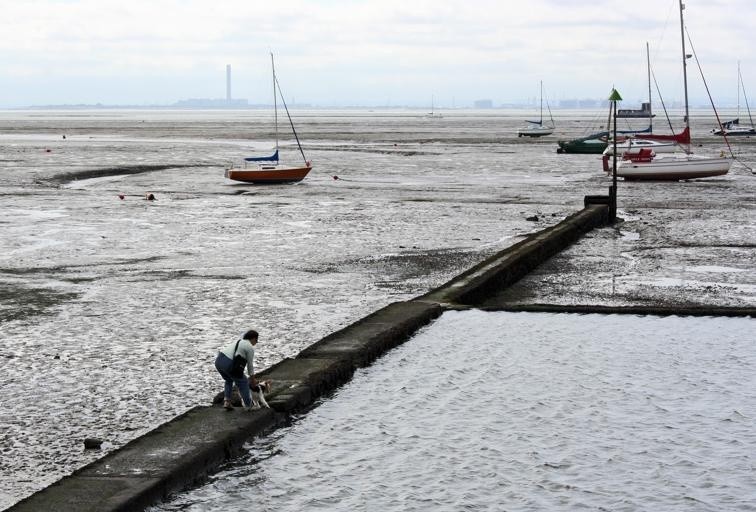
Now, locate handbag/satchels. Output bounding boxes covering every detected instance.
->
[232,355,248,378]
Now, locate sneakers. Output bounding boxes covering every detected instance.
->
[244,404,252,410]
[224,402,234,410]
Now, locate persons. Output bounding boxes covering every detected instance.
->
[212,330,260,412]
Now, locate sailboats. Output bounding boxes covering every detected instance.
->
[225,53,312,183]
[709,64,756,136]
[517,81,555,136]
[558,0,735,179]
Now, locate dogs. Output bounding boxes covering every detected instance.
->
[237,378,273,409]
